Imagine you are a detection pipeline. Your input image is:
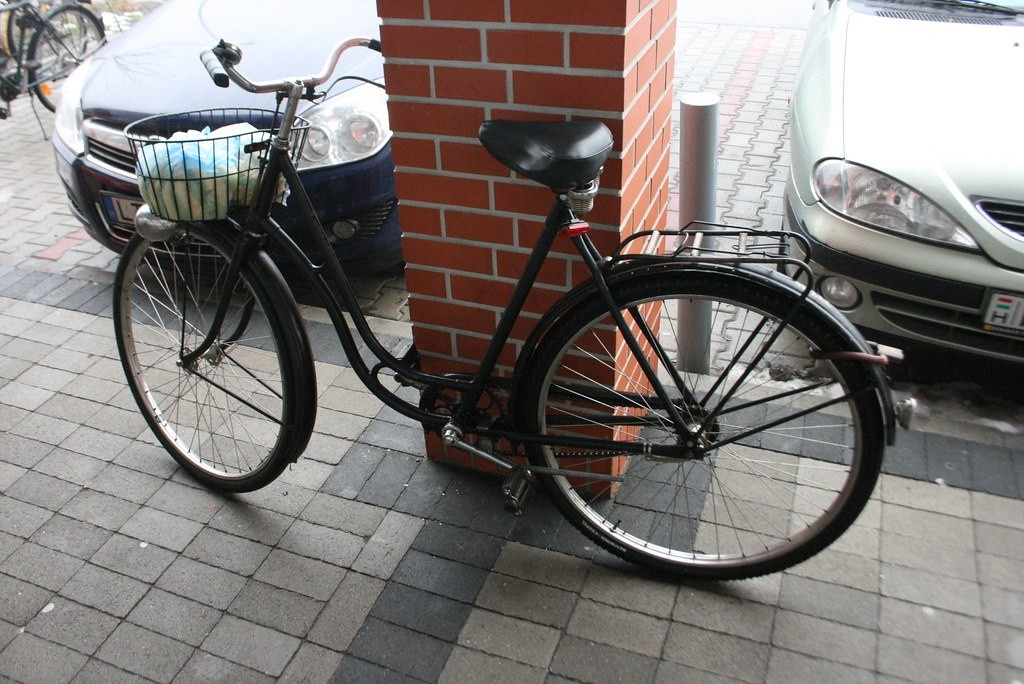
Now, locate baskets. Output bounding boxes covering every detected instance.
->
[125,107,313,221]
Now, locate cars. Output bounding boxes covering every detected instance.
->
[776,1,1024,380]
[52,0,409,276]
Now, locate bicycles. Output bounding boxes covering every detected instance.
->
[113,33,918,580]
[0,0,109,123]
